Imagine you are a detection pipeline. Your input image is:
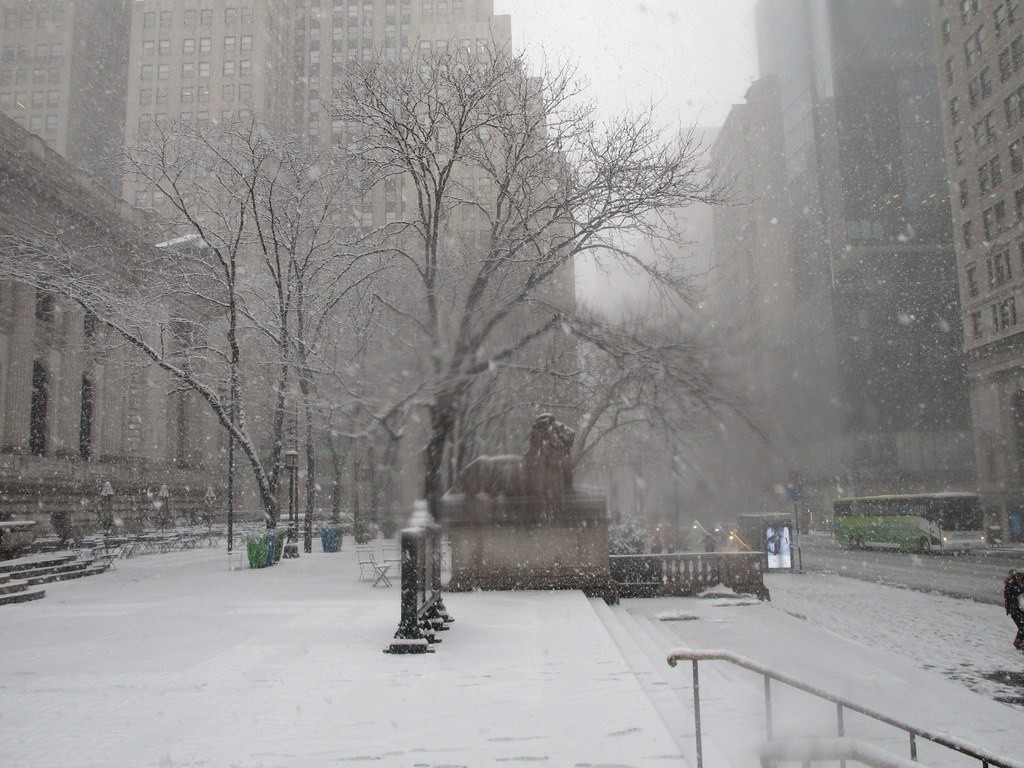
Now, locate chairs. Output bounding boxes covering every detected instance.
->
[381,542,403,579]
[356,543,378,582]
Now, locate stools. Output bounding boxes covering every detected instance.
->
[372,563,392,588]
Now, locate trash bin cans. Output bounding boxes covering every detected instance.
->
[321,524,344,552]
[247,528,288,569]
[987,526,1001,548]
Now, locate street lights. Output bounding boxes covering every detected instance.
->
[281,449,300,558]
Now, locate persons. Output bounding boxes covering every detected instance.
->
[1004,572,1024,651]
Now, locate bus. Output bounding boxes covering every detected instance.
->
[832,491,988,556]
